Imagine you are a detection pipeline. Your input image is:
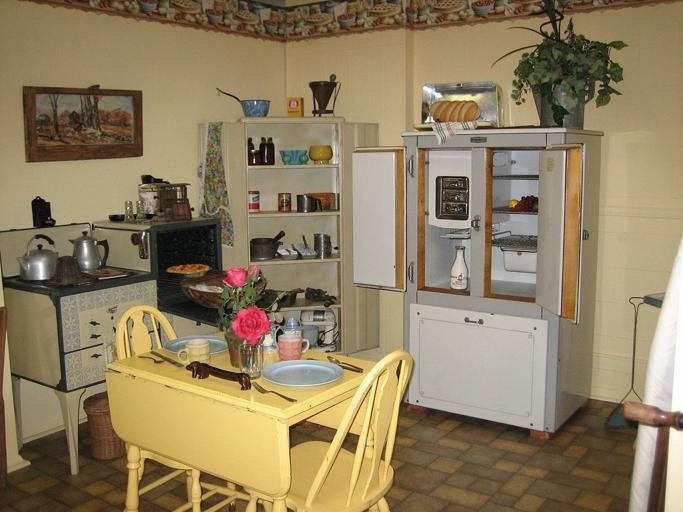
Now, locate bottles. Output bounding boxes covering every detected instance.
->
[448,246,468,291]
[123,201,147,223]
[247,135,274,166]
[274,318,301,350]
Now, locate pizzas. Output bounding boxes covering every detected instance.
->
[167,264,209,274]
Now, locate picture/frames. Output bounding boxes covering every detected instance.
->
[22,85,142,163]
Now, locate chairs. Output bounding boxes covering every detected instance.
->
[239,350,416,510]
[105,306,180,361]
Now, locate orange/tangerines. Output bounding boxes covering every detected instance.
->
[532,202,538,212]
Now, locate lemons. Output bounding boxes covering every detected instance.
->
[509,199,518,209]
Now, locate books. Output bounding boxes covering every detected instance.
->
[79,267,130,282]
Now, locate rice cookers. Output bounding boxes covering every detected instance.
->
[137,183,168,220]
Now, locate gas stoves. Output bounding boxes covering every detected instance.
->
[6,266,134,290]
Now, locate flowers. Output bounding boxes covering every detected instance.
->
[217,264,287,349]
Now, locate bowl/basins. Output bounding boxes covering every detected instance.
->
[279,149,306,165]
[308,145,332,161]
[240,99,270,117]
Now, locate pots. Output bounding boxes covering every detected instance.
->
[250,231,285,261]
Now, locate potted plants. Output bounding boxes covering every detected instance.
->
[490,0,630,131]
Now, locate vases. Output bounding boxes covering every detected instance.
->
[223,332,262,368]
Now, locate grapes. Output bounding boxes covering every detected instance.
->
[511,195,538,212]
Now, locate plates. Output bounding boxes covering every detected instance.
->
[165,264,212,279]
[163,336,228,355]
[262,359,343,386]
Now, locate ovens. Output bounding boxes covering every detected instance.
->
[91,218,224,309]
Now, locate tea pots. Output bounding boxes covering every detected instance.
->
[67,230,108,275]
[16,233,58,281]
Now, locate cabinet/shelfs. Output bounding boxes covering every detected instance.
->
[349,129,604,440]
[196,116,380,357]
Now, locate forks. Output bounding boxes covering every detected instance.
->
[138,356,164,364]
[251,381,297,403]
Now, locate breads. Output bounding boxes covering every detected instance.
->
[429,99,481,123]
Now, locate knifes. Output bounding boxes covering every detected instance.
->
[150,350,183,368]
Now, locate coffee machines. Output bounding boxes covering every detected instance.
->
[159,183,192,221]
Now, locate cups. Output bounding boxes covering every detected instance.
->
[238,343,262,379]
[176,339,210,365]
[278,335,309,361]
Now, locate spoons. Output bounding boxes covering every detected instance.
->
[326,356,363,371]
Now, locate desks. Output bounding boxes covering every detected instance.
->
[3,267,156,475]
